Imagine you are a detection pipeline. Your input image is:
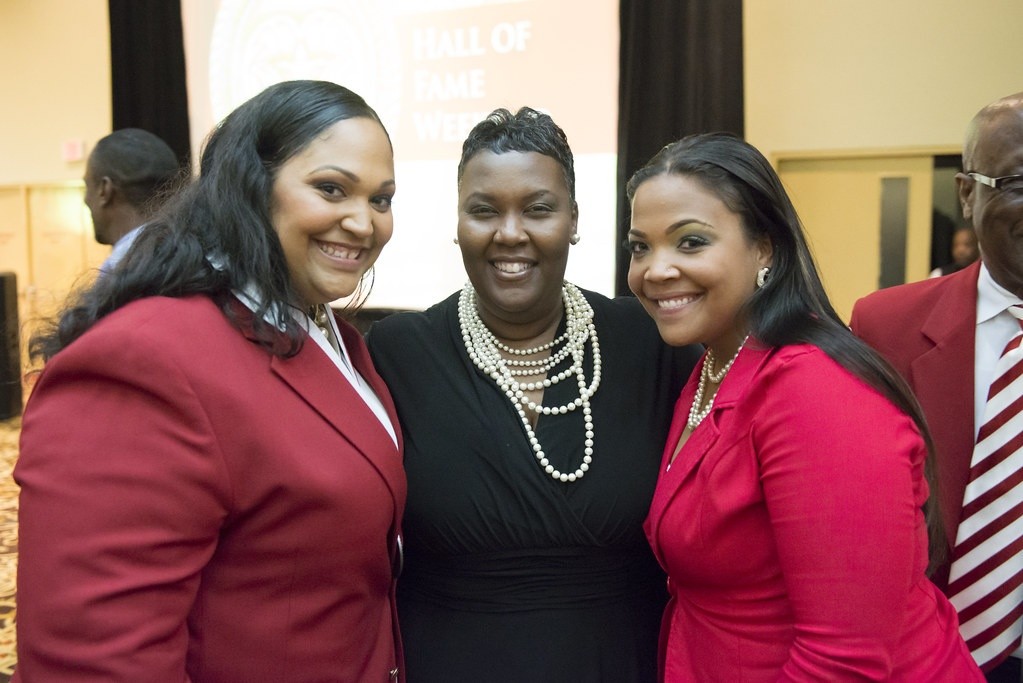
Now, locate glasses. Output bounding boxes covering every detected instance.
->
[967,172,1023,202]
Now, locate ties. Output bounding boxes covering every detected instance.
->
[947,305,1023,676]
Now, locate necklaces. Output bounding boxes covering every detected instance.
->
[457,278,601,482]
[687,330,752,430]
[317,310,329,339]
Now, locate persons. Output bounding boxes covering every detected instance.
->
[848,93,1023,683]
[361,106,706,683]
[626,131,986,683]
[929,227,980,279]
[82,128,179,285]
[12,80,408,683]
